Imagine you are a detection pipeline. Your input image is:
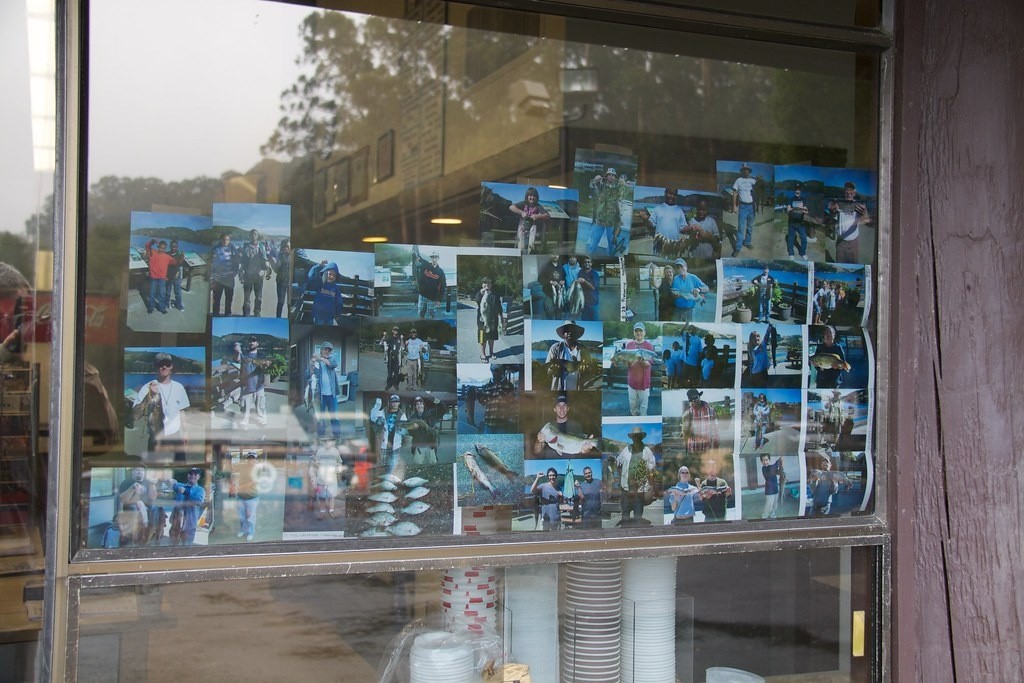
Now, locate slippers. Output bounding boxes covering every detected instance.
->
[480,352,497,363]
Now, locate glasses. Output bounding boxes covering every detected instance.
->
[687,396,699,402]
[156,362,172,367]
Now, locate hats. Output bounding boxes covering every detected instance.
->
[390,395,401,403]
[832,389,842,395]
[321,342,334,351]
[628,426,647,440]
[490,364,504,372]
[686,389,704,403]
[414,397,424,406]
[153,353,173,367]
[308,159,882,340]
[554,396,568,407]
[188,467,202,475]
[245,451,258,459]
[247,336,259,342]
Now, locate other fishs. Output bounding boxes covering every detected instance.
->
[780,470,788,506]
[826,395,845,432]
[517,219,537,254]
[810,353,851,373]
[590,183,625,253]
[246,355,438,466]
[538,423,602,455]
[652,231,699,259]
[137,500,190,546]
[810,467,853,491]
[612,349,658,367]
[770,327,778,369]
[670,288,707,306]
[357,475,433,536]
[147,391,164,448]
[464,443,520,498]
[552,281,585,314]
[684,332,691,359]
[745,179,868,226]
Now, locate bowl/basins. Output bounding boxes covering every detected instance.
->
[564,561,621,683]
[337,376,347,381]
[620,557,675,683]
[440,567,498,642]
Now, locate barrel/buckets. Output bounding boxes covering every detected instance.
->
[807,408,829,422]
[347,372,359,400]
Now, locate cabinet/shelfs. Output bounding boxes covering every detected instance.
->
[0,361,39,562]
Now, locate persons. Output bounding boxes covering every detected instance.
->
[689,197,720,259]
[112,336,272,548]
[411,244,447,319]
[786,182,810,261]
[475,277,506,364]
[752,265,775,324]
[210,227,291,318]
[732,161,760,257]
[613,325,850,527]
[811,279,849,325]
[826,182,870,265]
[658,258,711,322]
[538,255,601,321]
[306,327,439,521]
[587,167,627,256]
[477,321,604,530]
[145,237,186,314]
[0,261,121,683]
[640,188,696,258]
[308,258,342,327]
[509,187,551,254]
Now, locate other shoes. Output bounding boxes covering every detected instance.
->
[237,531,254,541]
[318,509,337,521]
[146,299,267,318]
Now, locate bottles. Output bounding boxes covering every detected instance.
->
[808,425,824,432]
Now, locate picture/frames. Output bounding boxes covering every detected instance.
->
[312,128,394,223]
